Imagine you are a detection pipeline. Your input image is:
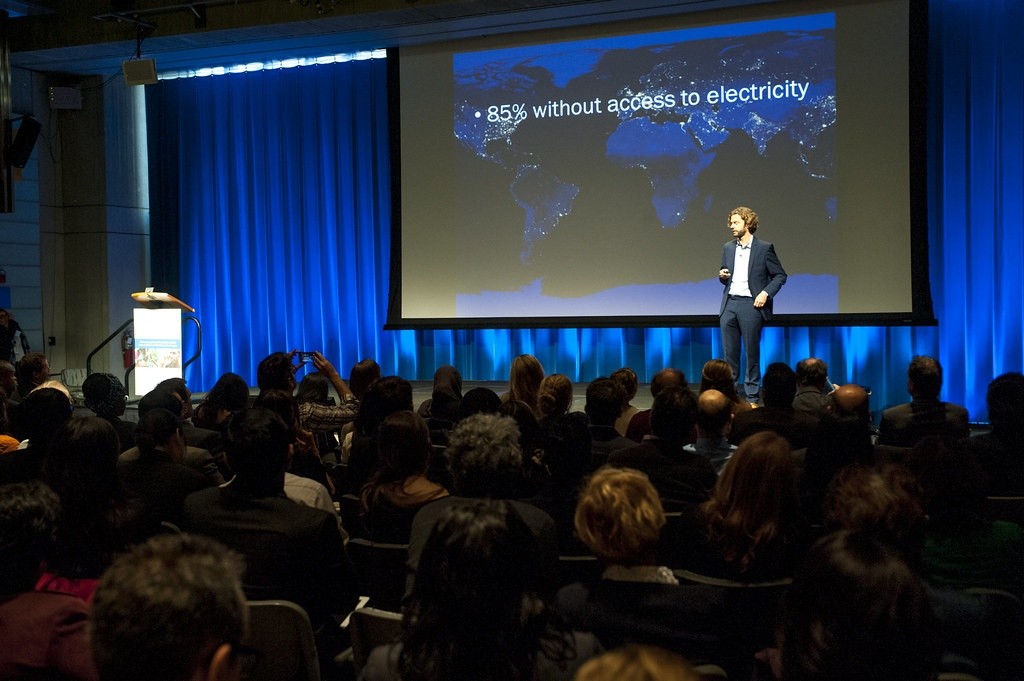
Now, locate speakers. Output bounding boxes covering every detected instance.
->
[8,116,41,168]
[123,58,160,87]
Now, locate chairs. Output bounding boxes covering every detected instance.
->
[158,443,1024,681]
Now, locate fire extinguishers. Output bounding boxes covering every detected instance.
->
[121,330,133,368]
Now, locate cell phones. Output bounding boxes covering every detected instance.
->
[299,351,317,363]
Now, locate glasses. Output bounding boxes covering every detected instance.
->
[67,404,76,411]
[290,364,298,381]
[201,642,266,681]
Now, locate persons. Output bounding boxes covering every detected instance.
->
[93,538,248,681]
[401,415,562,613]
[0,309,32,361]
[718,205,787,409]
[0,350,872,510]
[557,468,721,630]
[956,372,1024,496]
[88,490,152,550]
[0,514,120,681]
[753,534,949,681]
[0,481,67,560]
[181,412,340,582]
[575,646,698,681]
[676,432,802,568]
[354,501,601,681]
[873,356,969,450]
[816,454,933,578]
